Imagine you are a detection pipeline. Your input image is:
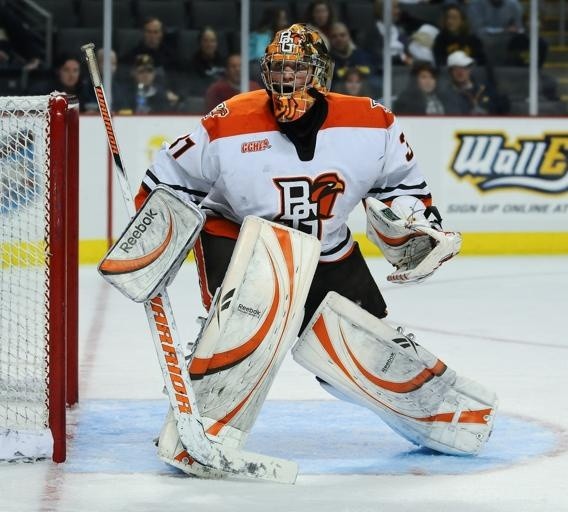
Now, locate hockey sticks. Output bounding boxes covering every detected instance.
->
[81,43,300,483]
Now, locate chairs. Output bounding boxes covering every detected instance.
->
[33,0,568,114]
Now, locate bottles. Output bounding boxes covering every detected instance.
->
[135,81,149,113]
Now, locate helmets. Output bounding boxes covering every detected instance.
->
[259,22,336,124]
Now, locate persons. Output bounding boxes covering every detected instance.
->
[27,0,560,117]
[98,21,497,479]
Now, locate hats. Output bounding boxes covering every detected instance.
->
[133,54,155,73]
[446,50,475,68]
